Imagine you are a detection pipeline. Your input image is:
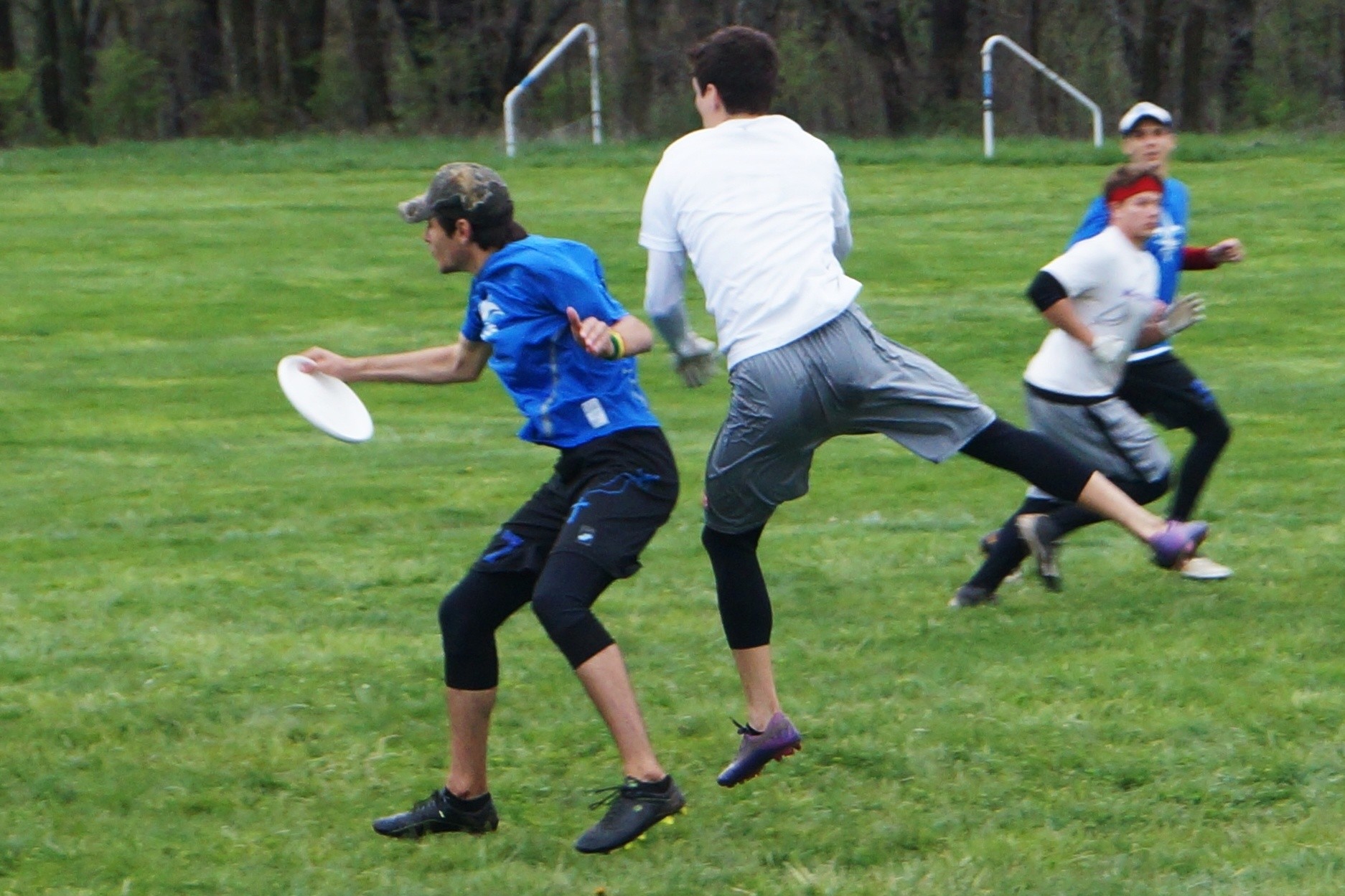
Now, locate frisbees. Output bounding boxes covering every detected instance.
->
[276,354,375,444]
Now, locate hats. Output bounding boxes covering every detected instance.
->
[399,161,513,225]
[1120,102,1173,132]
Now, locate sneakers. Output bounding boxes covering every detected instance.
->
[373,786,498,840]
[1150,517,1209,563]
[1179,556,1233,578]
[948,584,998,612]
[981,529,1022,584]
[1017,512,1065,593]
[717,711,801,787]
[576,776,684,854]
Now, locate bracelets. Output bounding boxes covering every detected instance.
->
[603,332,624,363]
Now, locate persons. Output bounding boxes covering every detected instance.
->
[299,162,685,856]
[637,25,1210,791]
[946,102,1247,605]
